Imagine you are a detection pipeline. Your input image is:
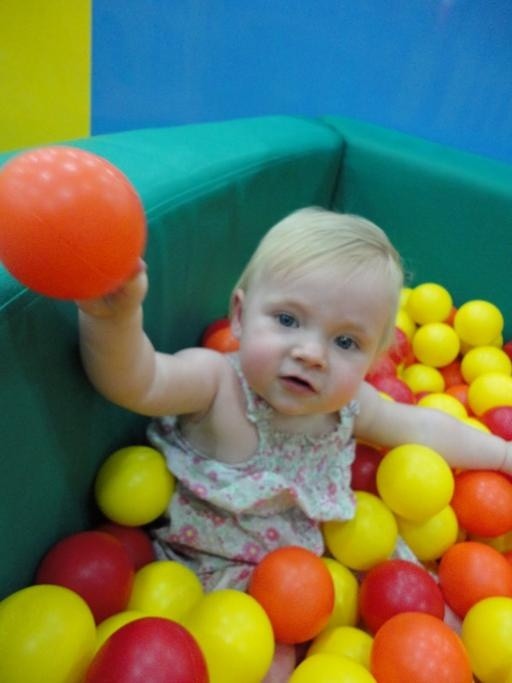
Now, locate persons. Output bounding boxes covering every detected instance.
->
[73,203,512,683]
